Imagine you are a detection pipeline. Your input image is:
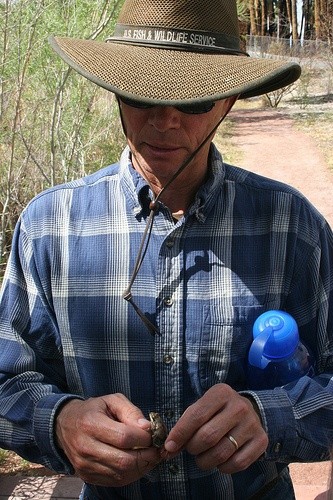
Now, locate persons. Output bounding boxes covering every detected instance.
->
[0,0,333,500]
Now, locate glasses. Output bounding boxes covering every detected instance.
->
[118,96,215,114]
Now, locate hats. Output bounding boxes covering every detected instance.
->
[46,0,302,107]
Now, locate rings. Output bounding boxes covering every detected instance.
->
[225,434,239,450]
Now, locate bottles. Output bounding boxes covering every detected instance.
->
[246,309,312,390]
